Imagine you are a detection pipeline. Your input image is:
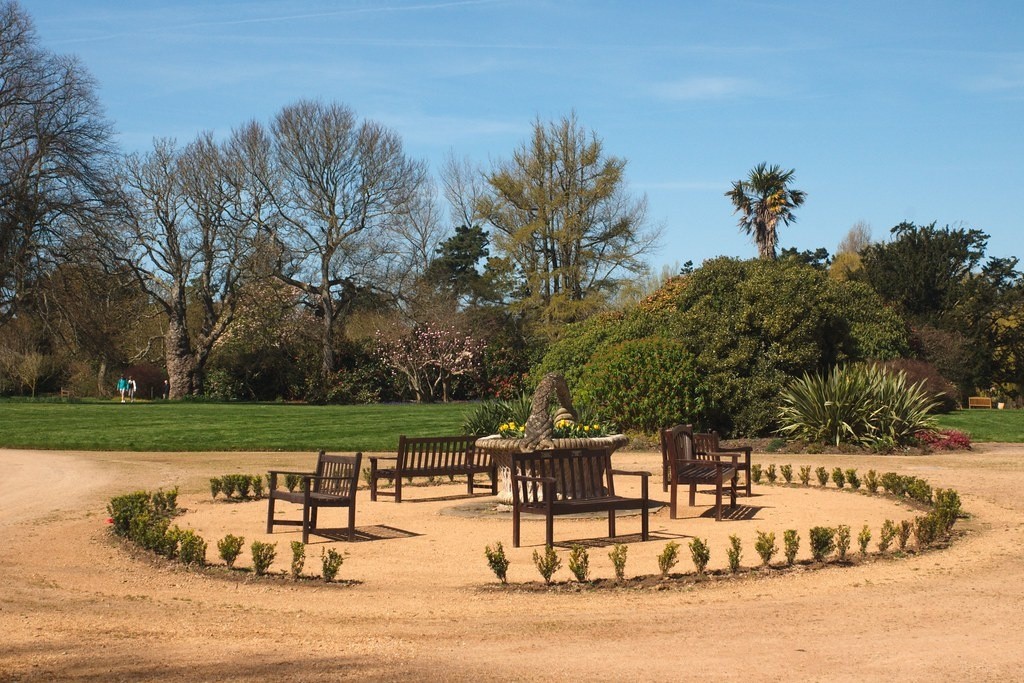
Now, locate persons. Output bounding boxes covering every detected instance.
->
[117,374,129,402]
[127,376,136,402]
[163,380,169,399]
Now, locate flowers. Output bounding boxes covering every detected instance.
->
[498,420,618,438]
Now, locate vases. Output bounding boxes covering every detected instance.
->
[475,434,629,506]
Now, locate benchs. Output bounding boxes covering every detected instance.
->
[368,435,498,503]
[659,427,754,497]
[510,446,653,550]
[666,424,742,522]
[266,450,362,544]
